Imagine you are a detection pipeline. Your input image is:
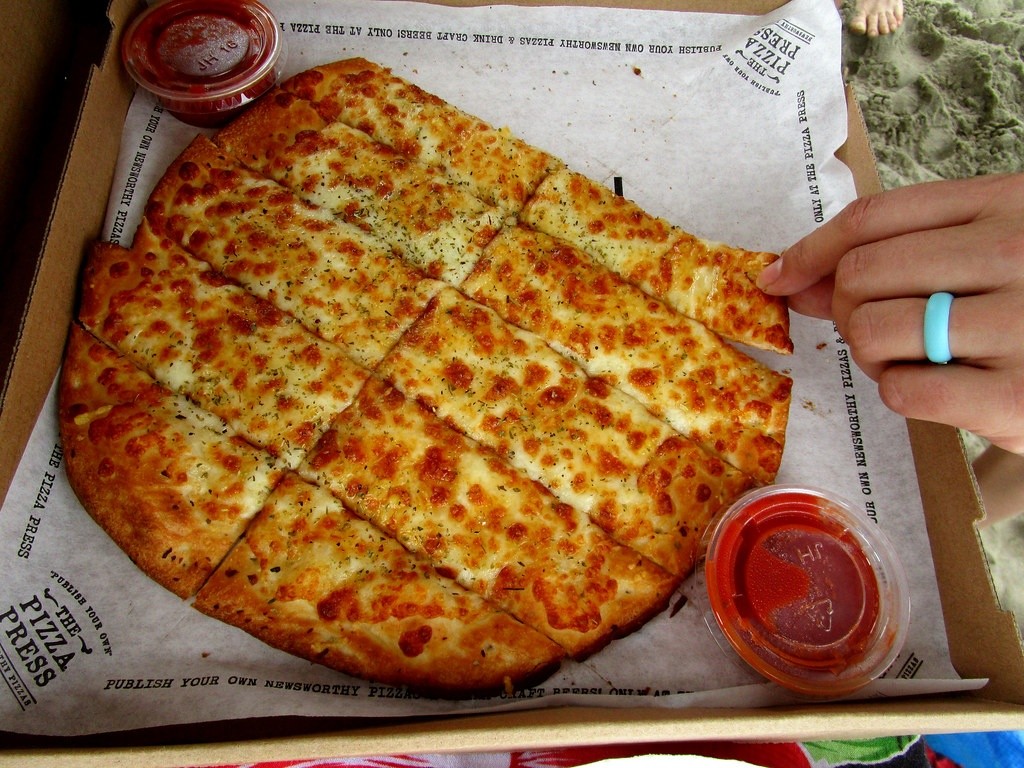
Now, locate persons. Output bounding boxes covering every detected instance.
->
[754,172,1024,531]
[849,0,904,36]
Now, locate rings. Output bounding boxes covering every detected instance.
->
[923,292,953,362]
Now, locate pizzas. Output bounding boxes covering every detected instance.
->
[56,56,795,688]
